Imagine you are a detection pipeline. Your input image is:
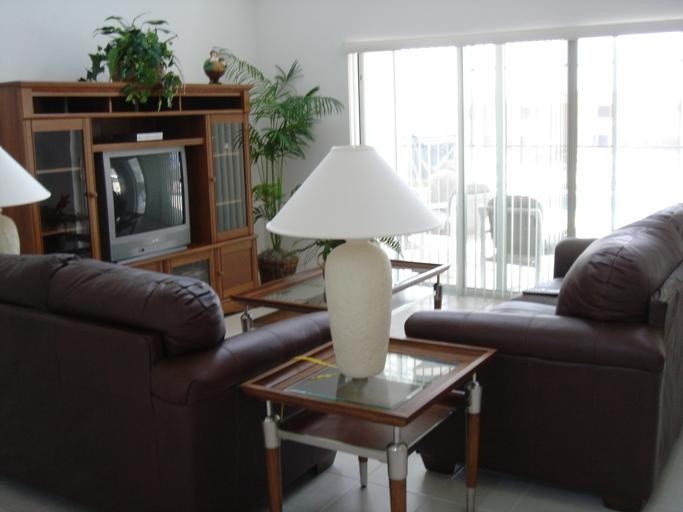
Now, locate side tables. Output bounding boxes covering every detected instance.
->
[239,335,498,511]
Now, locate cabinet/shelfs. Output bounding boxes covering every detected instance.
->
[0,81,259,318]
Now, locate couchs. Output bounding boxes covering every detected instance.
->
[1,254,337,510]
[405,203,682,512]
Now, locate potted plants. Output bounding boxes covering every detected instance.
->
[222,49,345,283]
[85,13,183,113]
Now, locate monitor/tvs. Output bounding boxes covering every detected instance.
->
[97,149,190,265]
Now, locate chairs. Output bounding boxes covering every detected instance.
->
[484,193,557,292]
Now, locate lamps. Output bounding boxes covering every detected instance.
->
[0,145,52,257]
[266,146,441,377]
